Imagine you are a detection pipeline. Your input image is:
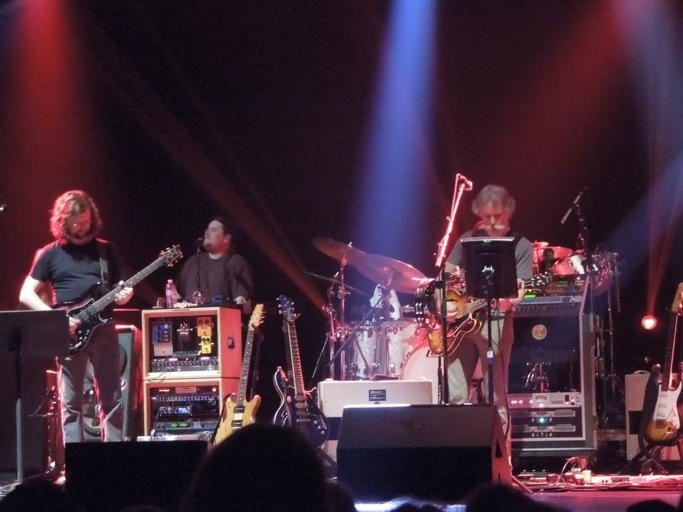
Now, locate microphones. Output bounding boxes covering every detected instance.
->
[195,237,203,257]
[458,174,474,191]
[559,183,590,224]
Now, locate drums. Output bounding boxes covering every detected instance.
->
[401,345,470,405]
[551,250,610,298]
[375,321,420,377]
[347,321,374,379]
[401,304,417,320]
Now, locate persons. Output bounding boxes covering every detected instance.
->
[435,185,534,470]
[18,190,134,448]
[175,216,257,315]
[0,421,683,511]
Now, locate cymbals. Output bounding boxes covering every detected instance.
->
[531,246,573,262]
[354,253,428,295]
[313,236,369,266]
[303,270,364,297]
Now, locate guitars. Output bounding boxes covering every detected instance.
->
[273,295,330,449]
[639,281,683,446]
[212,303,268,447]
[421,289,489,354]
[68,244,185,358]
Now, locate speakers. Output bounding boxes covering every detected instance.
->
[336,403,509,505]
[65,440,209,512]
[0,309,70,475]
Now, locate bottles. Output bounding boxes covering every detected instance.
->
[166,279,177,309]
